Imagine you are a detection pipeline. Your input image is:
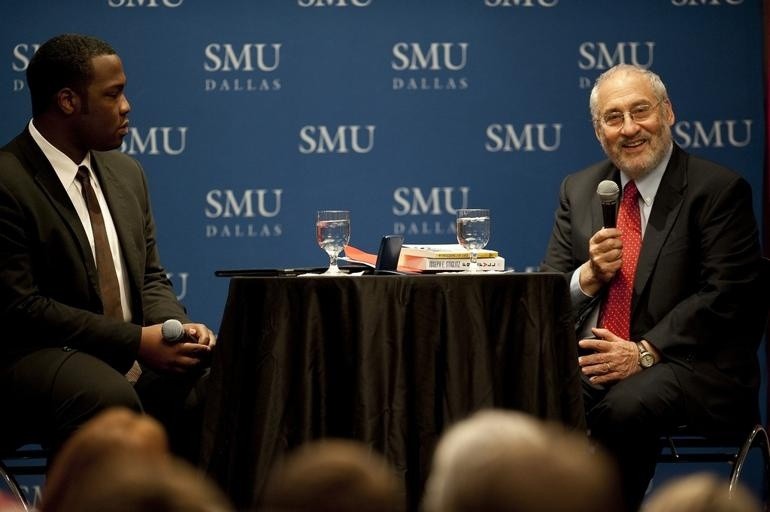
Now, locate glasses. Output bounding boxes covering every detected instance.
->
[595,99,663,128]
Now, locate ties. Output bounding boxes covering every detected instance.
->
[76,165,124,321]
[598,178,644,338]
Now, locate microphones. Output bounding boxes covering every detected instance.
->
[161,319,198,344]
[597,181,619,229]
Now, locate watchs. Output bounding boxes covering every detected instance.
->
[635,339,655,369]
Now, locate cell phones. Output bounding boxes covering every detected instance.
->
[375,235,402,271]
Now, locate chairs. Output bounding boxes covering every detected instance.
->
[657,423,770,501]
[0,442,53,508]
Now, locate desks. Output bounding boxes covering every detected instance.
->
[207,272,579,512]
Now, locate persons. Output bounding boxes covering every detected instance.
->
[262,440,410,512]
[39,407,166,511]
[421,408,613,512]
[0,34,216,487]
[54,446,228,512]
[544,63,766,502]
[641,471,762,512]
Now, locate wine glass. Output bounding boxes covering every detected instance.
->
[457,209,490,272]
[315,210,351,276]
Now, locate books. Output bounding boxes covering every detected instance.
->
[400,244,499,258]
[398,257,505,270]
